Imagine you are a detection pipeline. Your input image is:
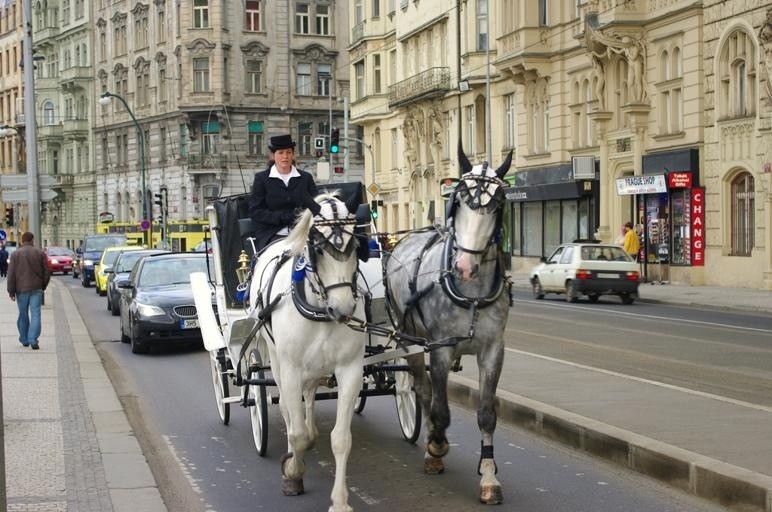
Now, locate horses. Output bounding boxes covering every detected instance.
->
[250,182,368,512]
[386,140,514,505]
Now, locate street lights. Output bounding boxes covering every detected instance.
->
[0,125,25,146]
[97,91,148,244]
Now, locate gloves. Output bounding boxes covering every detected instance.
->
[280,213,296,225]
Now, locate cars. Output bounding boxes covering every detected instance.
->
[529,241,642,305]
[117,251,215,355]
[3,240,21,271]
[42,232,148,296]
[103,248,172,316]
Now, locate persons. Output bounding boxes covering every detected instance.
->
[7,232,51,349]
[249,133,321,275]
[623,222,641,261]
[618,223,627,246]
[0,244,9,277]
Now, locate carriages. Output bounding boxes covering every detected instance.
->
[188,142,513,512]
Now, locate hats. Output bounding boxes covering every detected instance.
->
[269,135,295,152]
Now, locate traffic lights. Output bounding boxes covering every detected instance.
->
[313,138,326,158]
[159,215,163,230]
[328,127,341,154]
[155,193,162,205]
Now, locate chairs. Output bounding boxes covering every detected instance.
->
[237,203,371,259]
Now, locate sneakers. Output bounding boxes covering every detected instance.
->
[19,337,39,349]
